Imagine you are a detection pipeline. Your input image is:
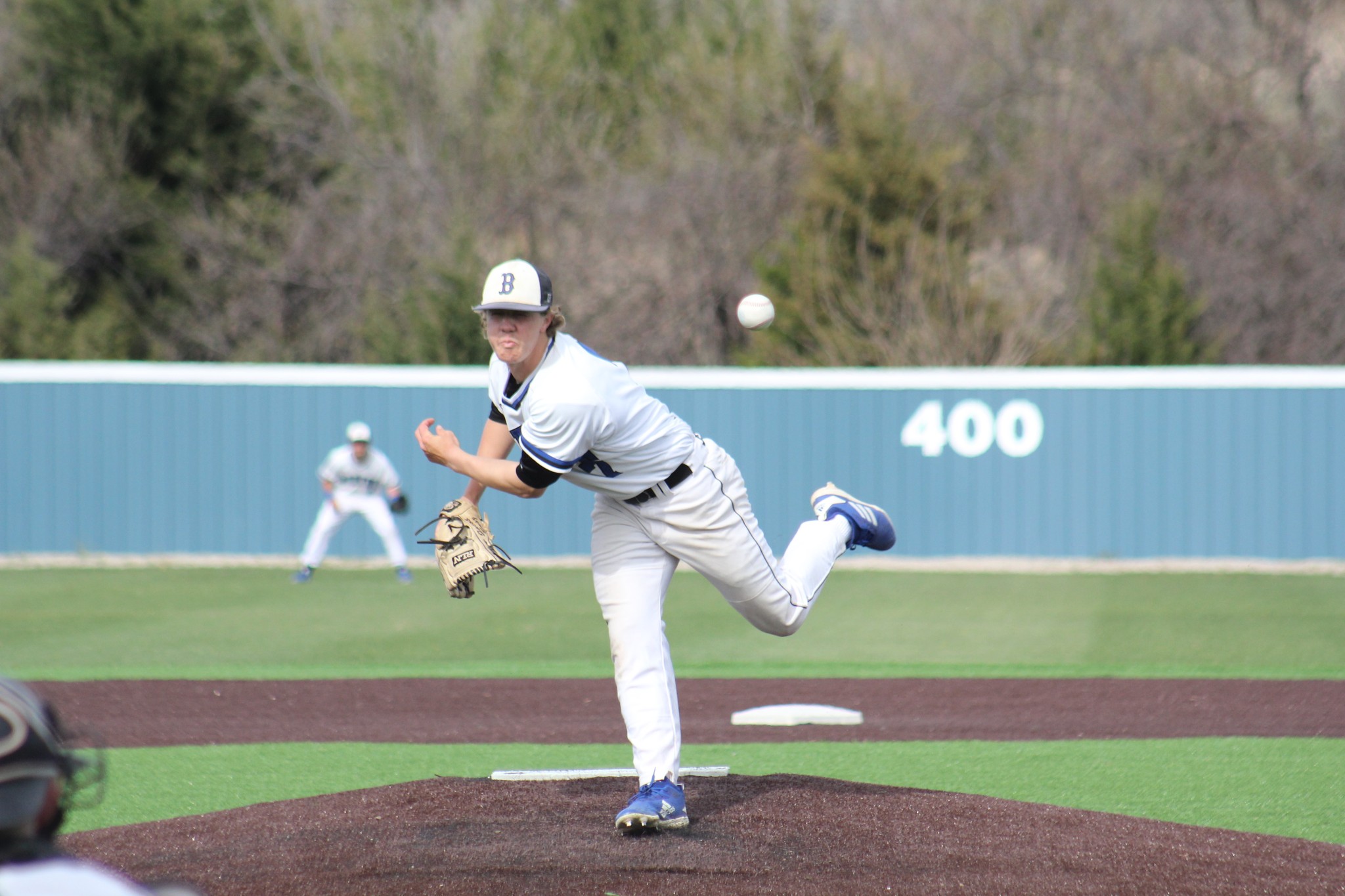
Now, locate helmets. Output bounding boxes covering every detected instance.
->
[0,678,107,841]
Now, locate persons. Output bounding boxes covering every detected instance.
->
[293,420,413,586]
[0,679,199,896]
[415,260,897,833]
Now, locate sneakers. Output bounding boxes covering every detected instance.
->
[615,767,689,836]
[810,482,896,550]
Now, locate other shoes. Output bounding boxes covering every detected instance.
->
[292,570,312,583]
[399,569,413,584]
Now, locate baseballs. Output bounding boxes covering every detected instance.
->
[736,294,775,332]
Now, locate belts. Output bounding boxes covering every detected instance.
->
[622,434,705,506]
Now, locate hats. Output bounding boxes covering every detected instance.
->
[347,422,371,442]
[471,259,553,311]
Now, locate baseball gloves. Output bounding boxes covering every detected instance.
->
[390,494,409,514]
[434,498,505,598]
